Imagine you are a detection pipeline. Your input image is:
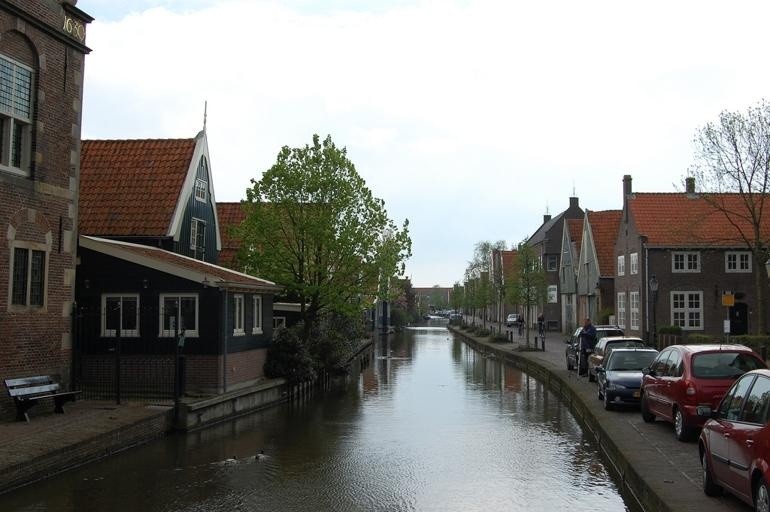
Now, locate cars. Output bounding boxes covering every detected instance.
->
[438,309,464,321]
[564,325,770,512]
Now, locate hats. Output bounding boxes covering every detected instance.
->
[584,316,591,320]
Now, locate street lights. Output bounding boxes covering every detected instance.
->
[648,274,660,349]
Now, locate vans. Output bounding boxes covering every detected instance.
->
[506,314,520,328]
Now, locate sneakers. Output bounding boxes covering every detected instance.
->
[581,372,588,377]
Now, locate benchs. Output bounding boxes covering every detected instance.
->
[4,375,83,423]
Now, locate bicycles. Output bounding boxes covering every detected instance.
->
[538,323,547,338]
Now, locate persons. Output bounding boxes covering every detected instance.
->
[537,312,545,336]
[515,314,525,336]
[577,318,597,375]
